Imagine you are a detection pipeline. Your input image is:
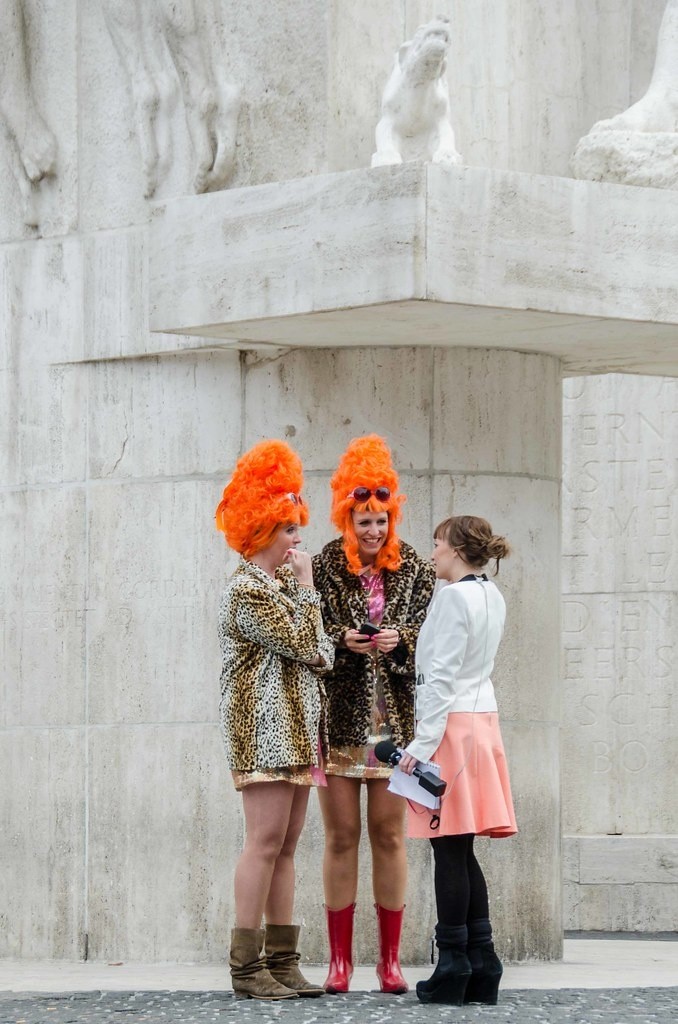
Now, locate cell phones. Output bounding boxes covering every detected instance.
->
[355,622,380,643]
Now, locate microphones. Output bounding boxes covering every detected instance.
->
[374,741,447,797]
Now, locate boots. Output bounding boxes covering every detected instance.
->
[415,923,472,1007]
[228,927,298,1000]
[373,902,409,993]
[263,923,326,995]
[321,901,354,993]
[463,918,503,1005]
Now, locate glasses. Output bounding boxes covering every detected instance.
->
[278,492,303,506]
[347,485,390,503]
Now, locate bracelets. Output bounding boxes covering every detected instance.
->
[297,583,316,590]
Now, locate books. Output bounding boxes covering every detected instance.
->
[387,747,441,810]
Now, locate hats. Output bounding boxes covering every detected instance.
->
[215,439,310,558]
[330,433,407,576]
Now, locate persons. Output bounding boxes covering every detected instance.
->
[399,516,519,1005]
[214,438,334,1000]
[309,432,438,992]
